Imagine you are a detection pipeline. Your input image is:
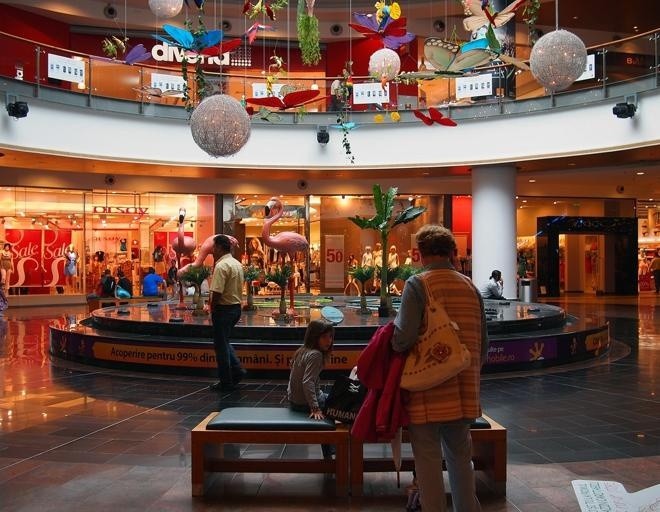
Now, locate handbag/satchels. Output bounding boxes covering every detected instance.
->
[399,302,472,392]
[324,365,367,424]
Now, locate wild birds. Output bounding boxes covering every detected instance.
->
[261,196,311,316]
[176,233,240,279]
[171,207,196,308]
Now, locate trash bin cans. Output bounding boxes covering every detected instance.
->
[518,278,537,303]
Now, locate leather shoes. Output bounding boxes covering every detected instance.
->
[232,369,247,385]
[210,383,233,391]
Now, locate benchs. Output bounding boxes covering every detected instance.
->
[190,407,506,501]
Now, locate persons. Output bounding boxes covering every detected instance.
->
[387,224,489,512]
[479,270,507,300]
[205,235,249,392]
[343,242,412,296]
[101,244,178,305]
[286,319,336,479]
[250,238,265,255]
[327,73,385,111]
[517,249,529,278]
[647,251,660,293]
[637,250,647,279]
[64,244,81,295]
[0,243,15,295]
